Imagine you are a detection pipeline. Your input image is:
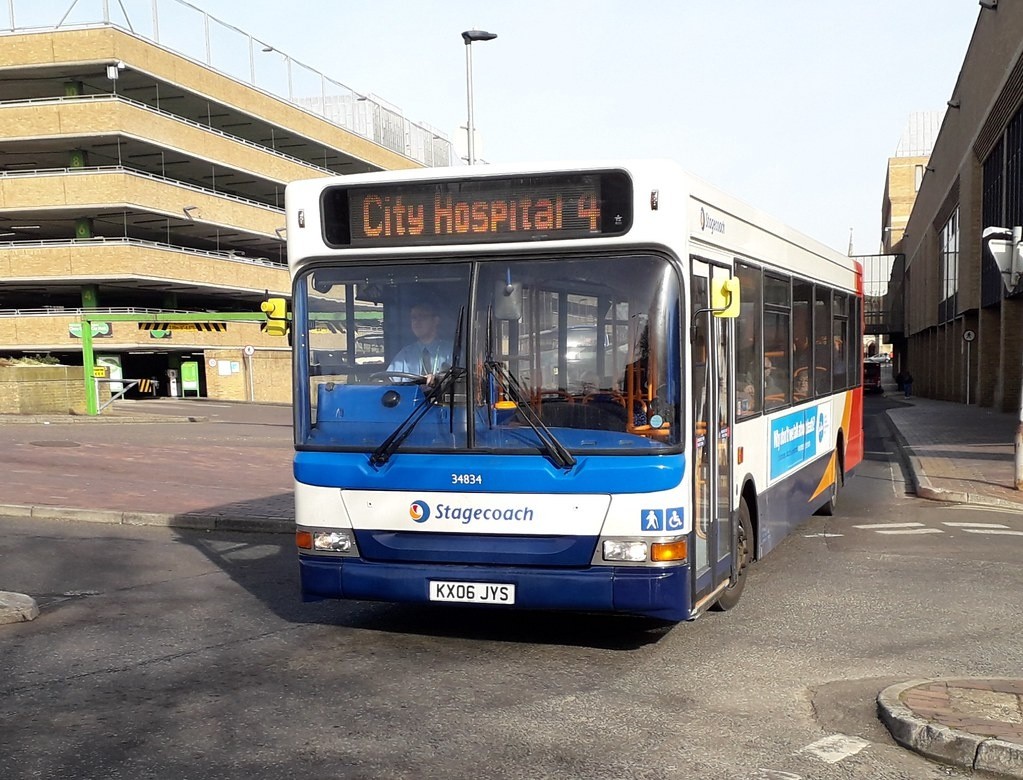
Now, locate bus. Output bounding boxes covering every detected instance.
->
[261,155,863,622]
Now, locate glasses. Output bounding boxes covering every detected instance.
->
[407,314,436,322]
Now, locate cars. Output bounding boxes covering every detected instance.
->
[868,352,891,363]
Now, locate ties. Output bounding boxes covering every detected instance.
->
[421,347,432,376]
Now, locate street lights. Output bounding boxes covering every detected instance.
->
[458,29,498,161]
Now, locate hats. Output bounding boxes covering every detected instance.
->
[834,336,842,344]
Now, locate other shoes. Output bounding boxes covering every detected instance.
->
[907,397,911,400]
[905,397,907,399]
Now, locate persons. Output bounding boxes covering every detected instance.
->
[743,356,818,396]
[386,296,454,385]
[903,371,913,400]
